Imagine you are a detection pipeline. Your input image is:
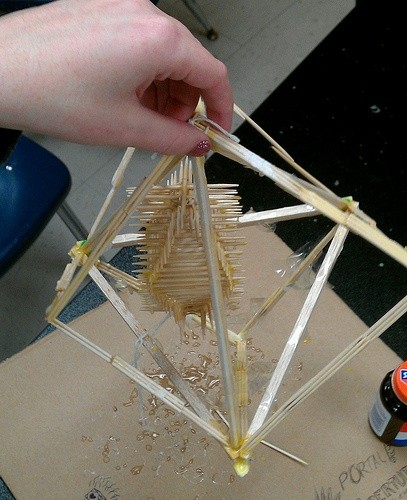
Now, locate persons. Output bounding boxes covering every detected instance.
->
[0,0,235,159]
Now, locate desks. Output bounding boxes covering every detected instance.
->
[0,0,406,500]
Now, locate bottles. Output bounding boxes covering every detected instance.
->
[366,360,407,449]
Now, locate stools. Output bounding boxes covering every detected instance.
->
[0,127,107,283]
